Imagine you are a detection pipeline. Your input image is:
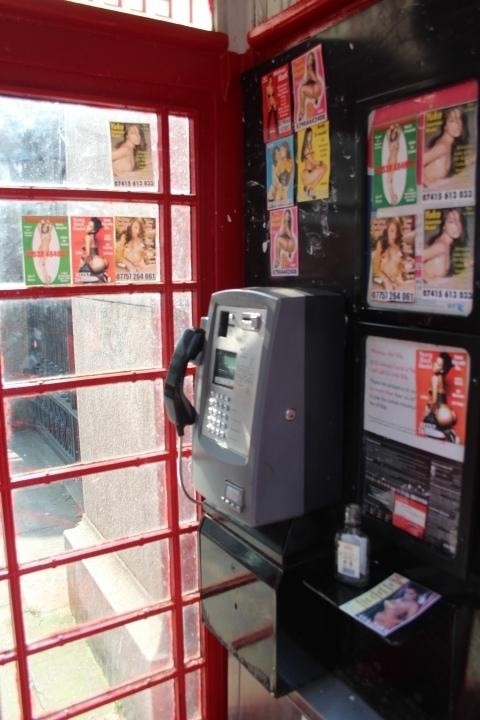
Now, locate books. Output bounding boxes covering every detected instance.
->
[341,572,443,638]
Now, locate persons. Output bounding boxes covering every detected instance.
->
[277,141,293,201]
[374,583,420,629]
[372,217,415,294]
[423,208,471,290]
[385,122,402,204]
[36,218,53,284]
[273,209,297,267]
[421,106,469,191]
[111,125,149,179]
[297,53,323,121]
[117,217,149,274]
[267,146,283,202]
[300,126,326,200]
[265,76,279,140]
[81,218,112,284]
[420,351,461,443]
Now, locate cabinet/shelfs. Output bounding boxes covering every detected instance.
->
[196,35,478,718]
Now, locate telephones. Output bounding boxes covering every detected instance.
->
[162,286,345,529]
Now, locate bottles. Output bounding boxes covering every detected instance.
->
[334,503,372,587]
